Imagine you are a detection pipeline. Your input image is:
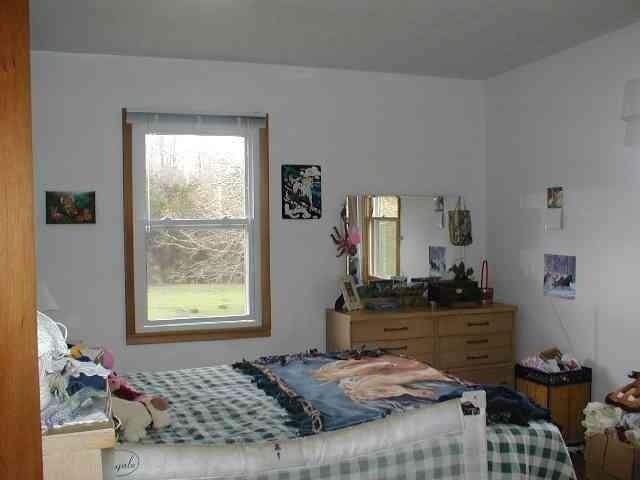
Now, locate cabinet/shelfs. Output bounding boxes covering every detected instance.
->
[326,300,518,389]
[515,359,592,450]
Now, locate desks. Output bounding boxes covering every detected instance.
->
[40,416,118,479]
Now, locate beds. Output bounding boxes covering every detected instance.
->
[36,307,576,478]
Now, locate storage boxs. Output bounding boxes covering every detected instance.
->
[582,433,640,480]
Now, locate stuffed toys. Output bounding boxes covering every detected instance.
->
[91,343,172,444]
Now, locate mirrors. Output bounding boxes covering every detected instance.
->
[346,191,400,286]
